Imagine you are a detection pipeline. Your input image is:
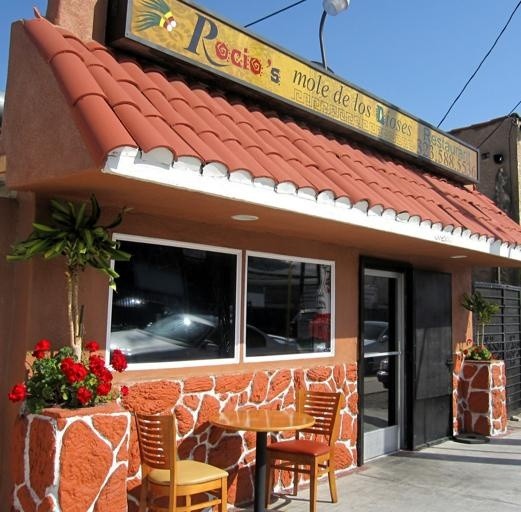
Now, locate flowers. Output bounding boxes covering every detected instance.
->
[6,341,134,414]
[461,337,496,361]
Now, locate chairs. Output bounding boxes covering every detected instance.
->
[133,406,230,512]
[263,383,348,512]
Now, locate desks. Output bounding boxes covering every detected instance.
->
[210,409,315,512]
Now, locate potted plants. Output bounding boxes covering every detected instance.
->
[3,191,139,510]
[458,287,509,438]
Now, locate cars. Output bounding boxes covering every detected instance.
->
[290,309,318,341]
[109,313,303,364]
[377,358,389,387]
[314,321,391,371]
[111,294,183,332]
